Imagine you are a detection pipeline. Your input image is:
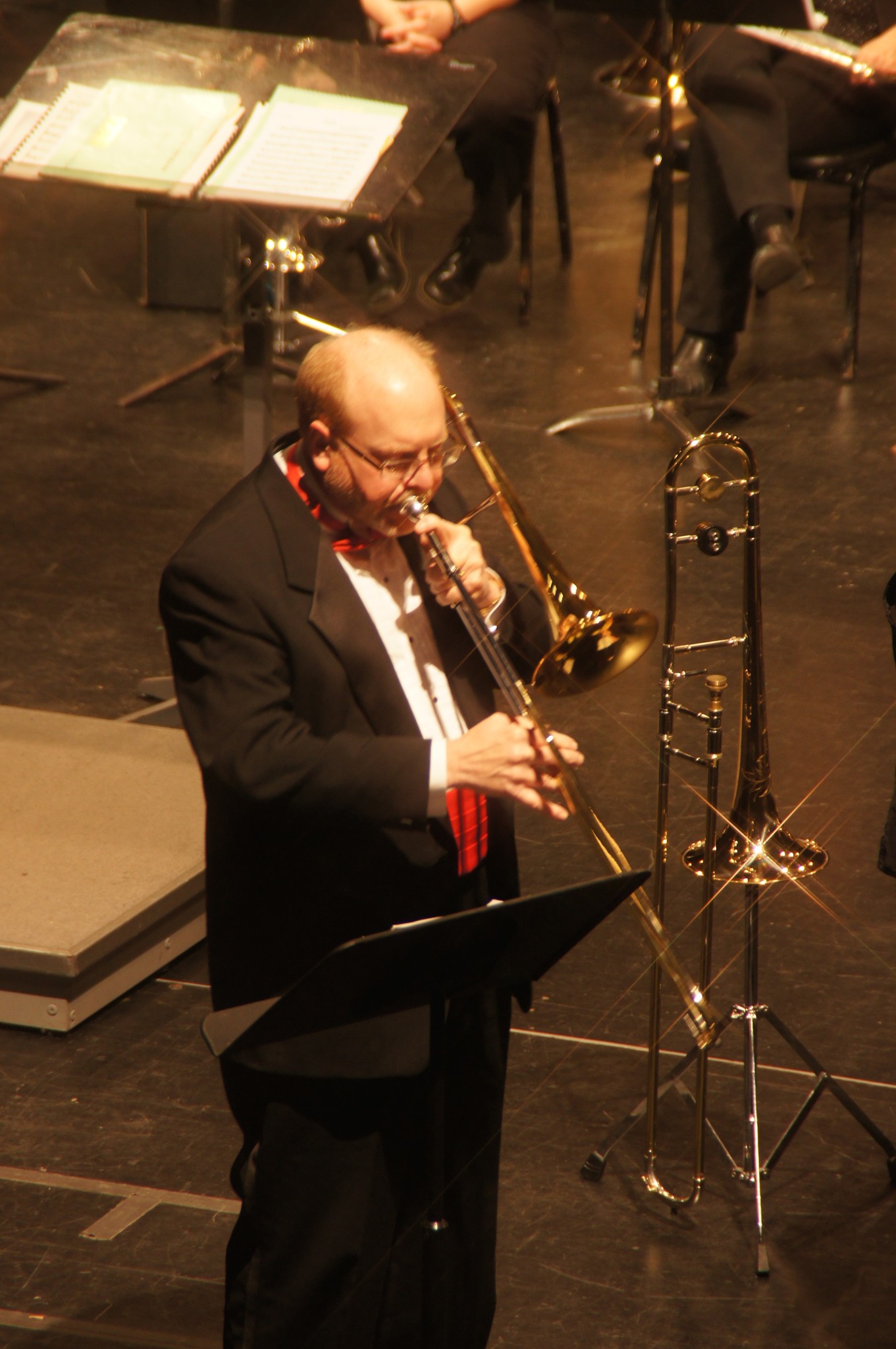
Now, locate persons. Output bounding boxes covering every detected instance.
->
[353,0,545,306]
[158,323,585,1349]
[673,2,896,397]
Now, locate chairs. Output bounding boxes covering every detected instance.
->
[507,72,573,325]
[629,111,896,383]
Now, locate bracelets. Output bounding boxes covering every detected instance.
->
[448,0,466,37]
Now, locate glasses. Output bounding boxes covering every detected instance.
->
[322,423,465,480]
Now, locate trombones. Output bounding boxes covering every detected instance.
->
[394,383,724,1048]
[638,429,831,1216]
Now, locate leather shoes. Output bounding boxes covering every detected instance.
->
[424,225,513,306]
[672,331,738,396]
[353,216,411,314]
[750,238,801,291]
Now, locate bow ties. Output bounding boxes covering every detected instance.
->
[284,444,384,553]
